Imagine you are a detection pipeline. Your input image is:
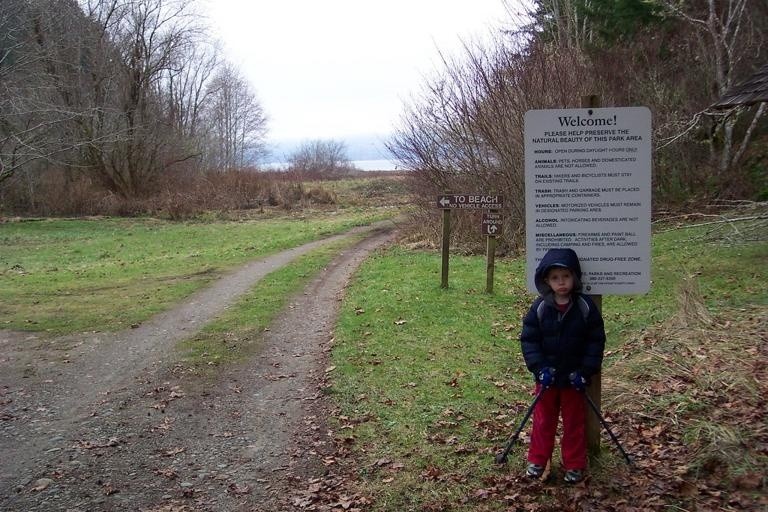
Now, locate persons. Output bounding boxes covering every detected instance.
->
[521,248,606,483]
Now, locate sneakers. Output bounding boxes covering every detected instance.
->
[527,464,545,477]
[564,469,582,482]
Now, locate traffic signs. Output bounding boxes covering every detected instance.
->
[437,194,505,210]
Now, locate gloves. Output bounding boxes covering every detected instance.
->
[569,371,592,394]
[536,366,556,389]
